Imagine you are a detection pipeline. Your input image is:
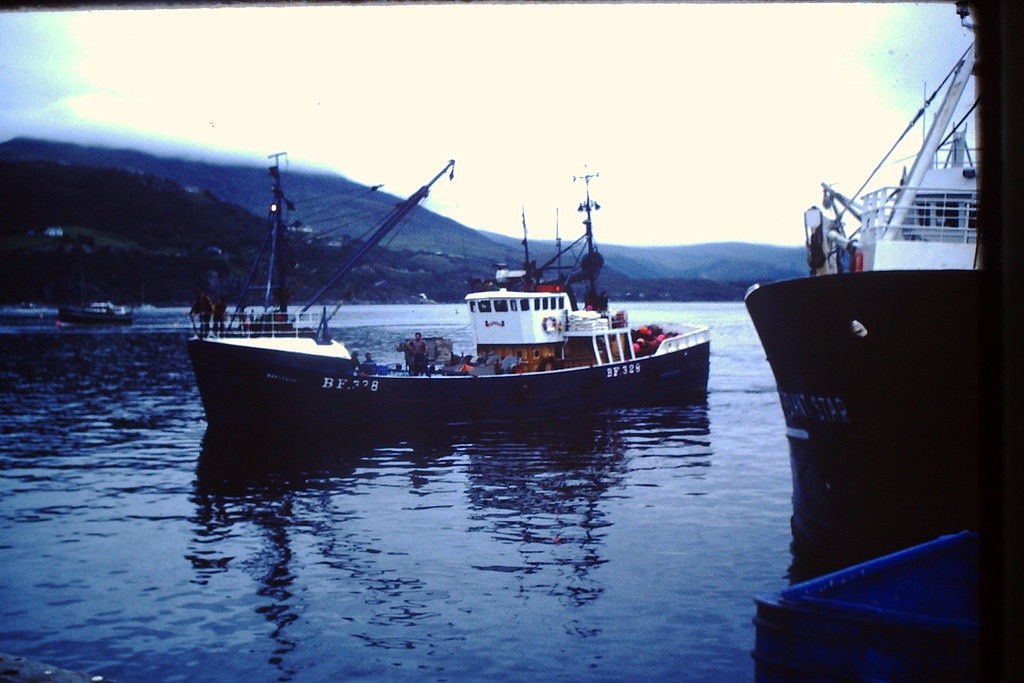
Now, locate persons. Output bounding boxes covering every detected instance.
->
[598,291,608,310]
[410,333,431,376]
[190,290,213,338]
[352,352,360,371]
[213,296,226,337]
[238,301,246,330]
[522,275,536,291]
[362,353,376,375]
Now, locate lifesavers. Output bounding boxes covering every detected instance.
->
[542,316,557,333]
[853,252,863,271]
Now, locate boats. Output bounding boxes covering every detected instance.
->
[58,301,135,326]
[743,0,1024,683]
[185,152,710,443]
